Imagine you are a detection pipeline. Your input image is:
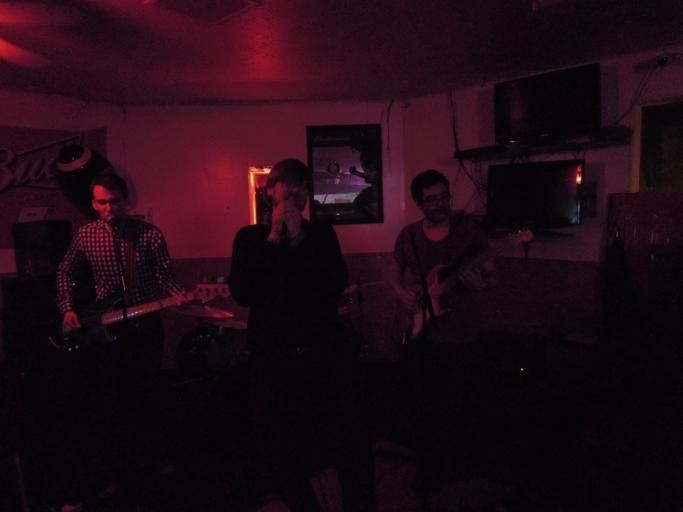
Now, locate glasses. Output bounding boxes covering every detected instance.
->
[421,192,449,203]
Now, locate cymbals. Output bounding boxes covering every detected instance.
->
[344,282,385,294]
[171,306,236,320]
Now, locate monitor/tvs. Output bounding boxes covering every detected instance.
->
[486,159,584,228]
[494,62,602,144]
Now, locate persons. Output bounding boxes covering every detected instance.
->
[228,158,375,509]
[55,167,190,512]
[385,168,500,460]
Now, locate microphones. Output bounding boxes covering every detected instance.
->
[107,215,120,238]
[277,196,297,243]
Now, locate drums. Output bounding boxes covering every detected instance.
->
[200,320,248,372]
[340,305,363,371]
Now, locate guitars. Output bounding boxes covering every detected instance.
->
[48,277,231,356]
[397,227,536,336]
